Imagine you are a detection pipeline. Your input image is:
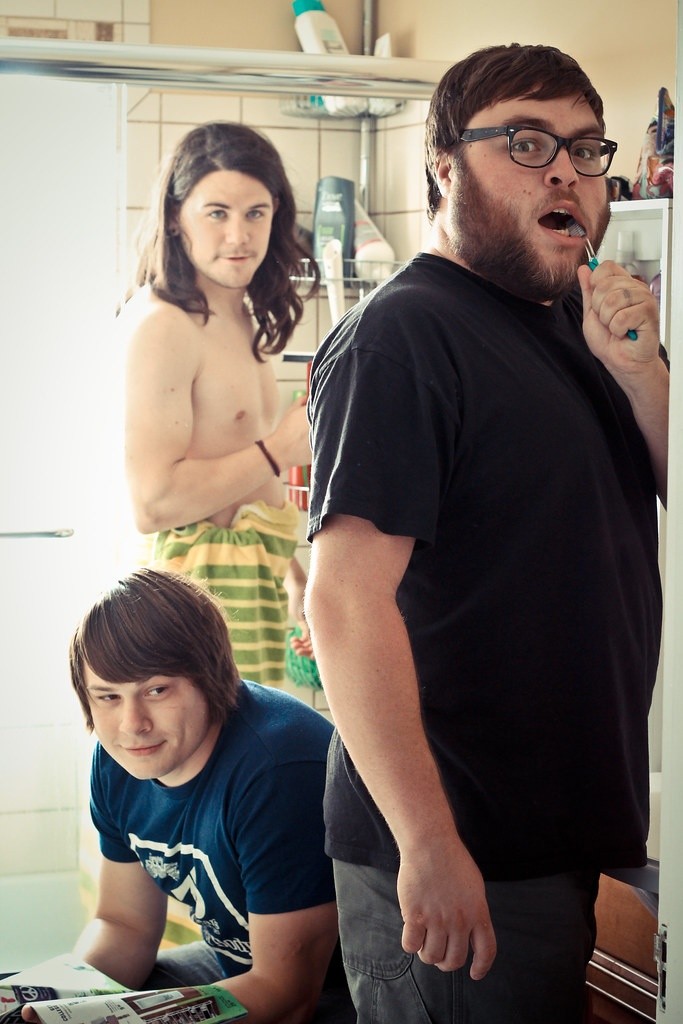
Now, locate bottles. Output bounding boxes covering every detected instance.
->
[612,230,647,285]
[289,391,311,512]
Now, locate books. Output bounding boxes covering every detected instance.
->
[0,952,249,1024]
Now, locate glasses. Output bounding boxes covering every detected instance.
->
[453,127,618,176]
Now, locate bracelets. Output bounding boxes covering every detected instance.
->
[256,440,282,477]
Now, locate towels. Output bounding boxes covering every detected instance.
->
[147,500,301,690]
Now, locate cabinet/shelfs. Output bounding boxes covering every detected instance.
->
[277,93,407,513]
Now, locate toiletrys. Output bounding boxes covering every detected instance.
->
[290,0,397,118]
[288,361,312,513]
[293,176,396,283]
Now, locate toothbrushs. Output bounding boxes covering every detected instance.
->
[568,222,662,341]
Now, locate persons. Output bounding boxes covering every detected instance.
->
[1,570,354,1024]
[298,41,669,1023]
[111,124,312,954]
